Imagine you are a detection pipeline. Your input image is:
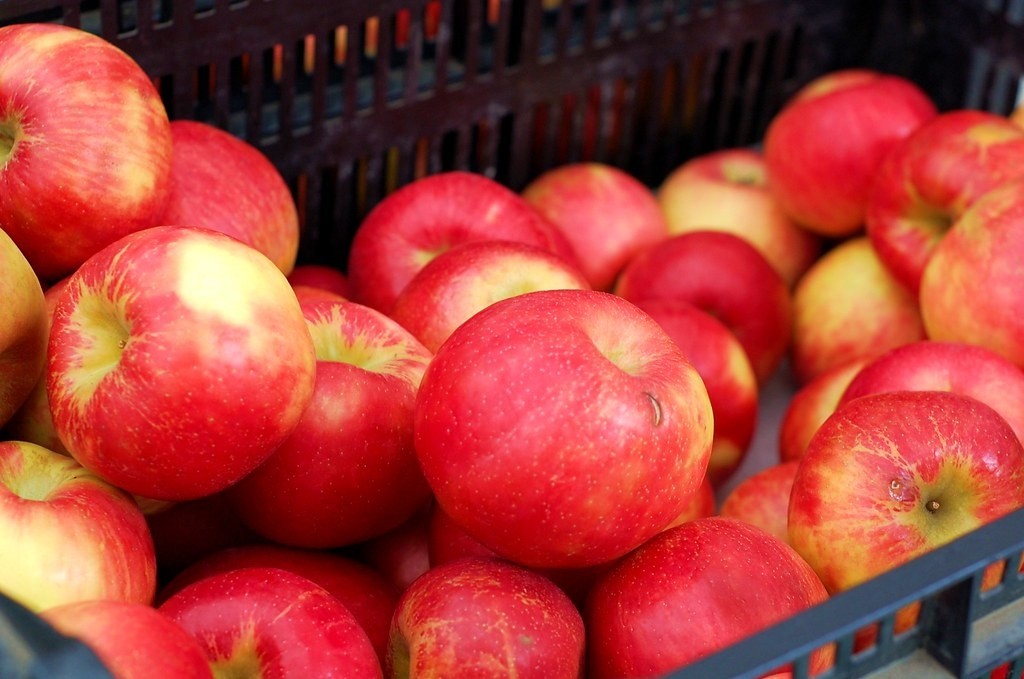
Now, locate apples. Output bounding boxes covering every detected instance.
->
[0,22,1024,678]
